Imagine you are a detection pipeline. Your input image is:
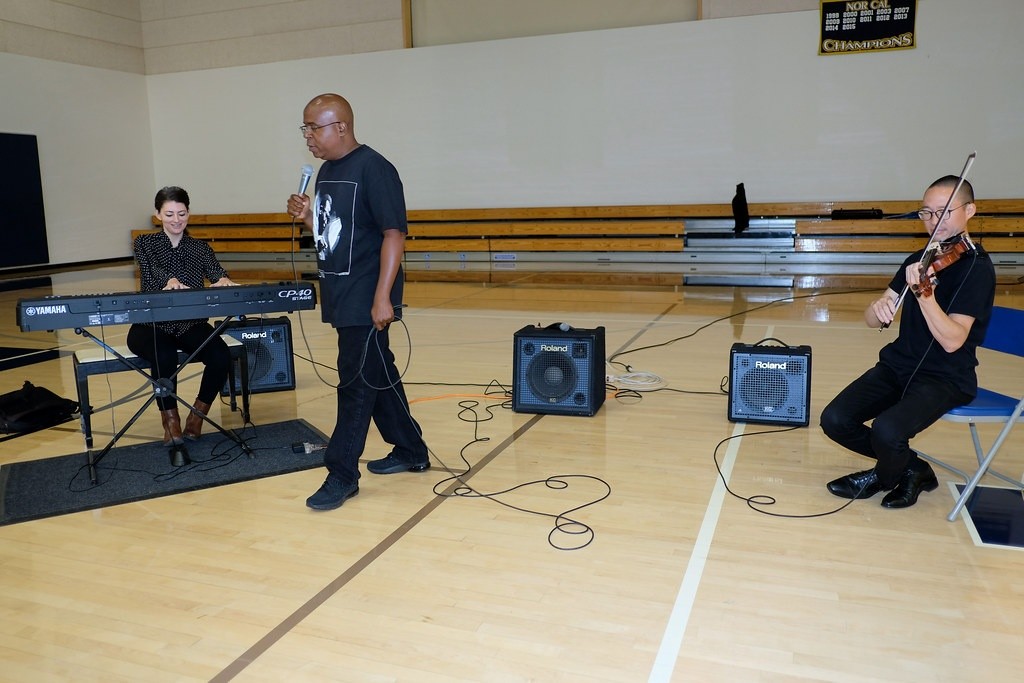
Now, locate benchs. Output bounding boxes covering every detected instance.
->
[71,333,250,448]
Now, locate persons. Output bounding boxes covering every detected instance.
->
[287,93,430,511]
[127,186,241,446]
[821,176,996,510]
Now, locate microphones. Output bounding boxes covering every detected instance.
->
[297,164,314,197]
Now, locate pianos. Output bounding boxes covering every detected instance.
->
[13,279,320,485]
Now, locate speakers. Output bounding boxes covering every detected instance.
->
[215,315,296,397]
[513,322,607,417]
[728,343,812,427]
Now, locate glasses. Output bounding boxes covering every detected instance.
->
[300,122,341,133]
[918,202,970,221]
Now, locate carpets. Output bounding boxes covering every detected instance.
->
[0,420,331,526]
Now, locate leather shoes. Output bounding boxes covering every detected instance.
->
[881,462,938,508]
[827,468,894,500]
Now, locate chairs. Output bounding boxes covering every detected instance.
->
[910,306,1024,523]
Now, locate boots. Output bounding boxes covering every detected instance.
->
[161,408,184,445]
[183,398,211,441]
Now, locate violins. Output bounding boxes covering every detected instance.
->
[912,230,974,300]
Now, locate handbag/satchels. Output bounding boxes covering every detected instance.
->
[0,384,76,432]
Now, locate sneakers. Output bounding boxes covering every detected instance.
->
[305,473,360,509]
[367,452,430,474]
